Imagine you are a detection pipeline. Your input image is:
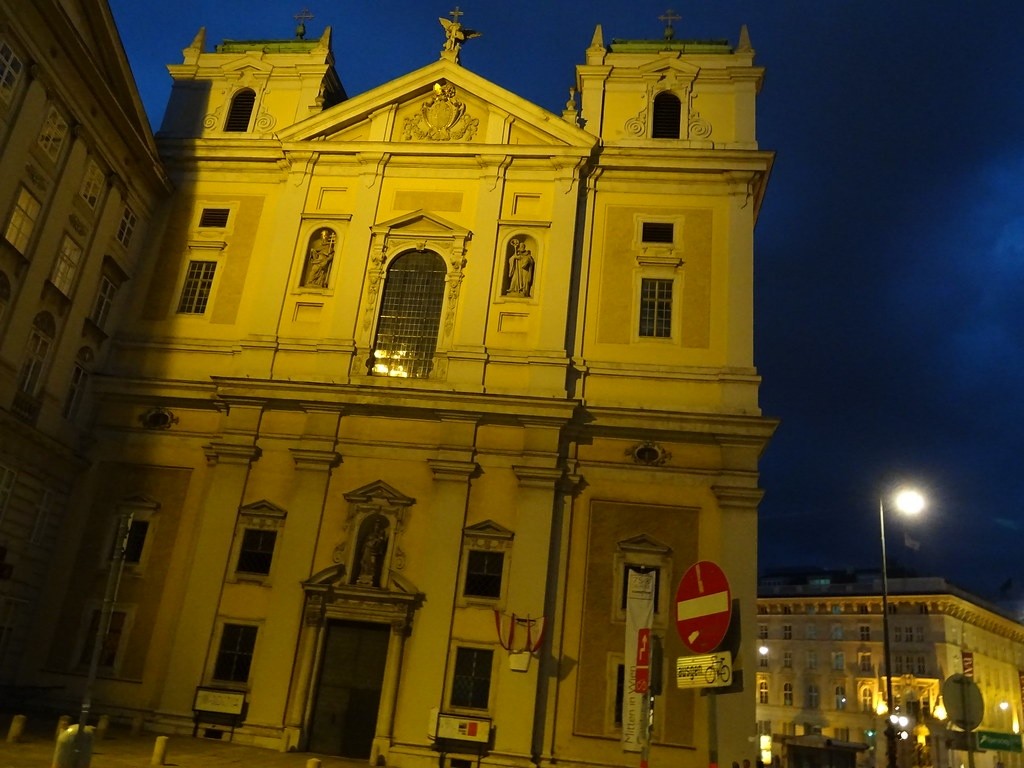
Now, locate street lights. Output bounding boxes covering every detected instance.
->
[878,482,927,768]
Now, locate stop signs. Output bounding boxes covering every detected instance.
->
[673,561,732,654]
